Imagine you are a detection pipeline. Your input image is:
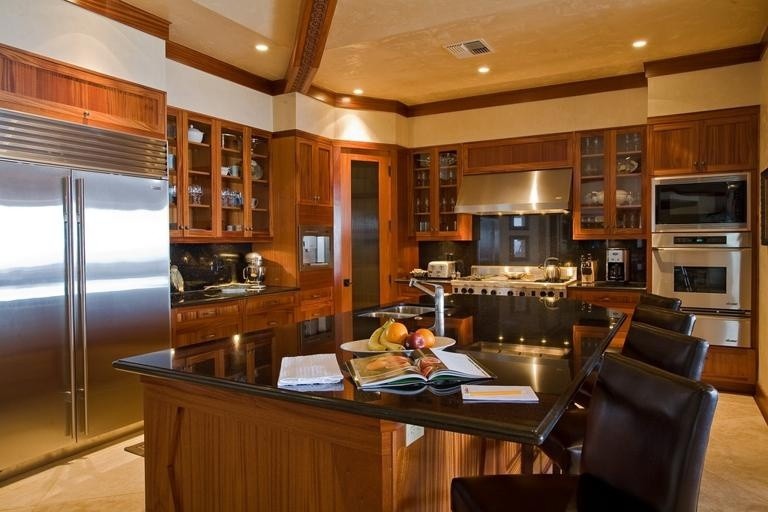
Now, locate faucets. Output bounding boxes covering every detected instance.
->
[408,276,446,336]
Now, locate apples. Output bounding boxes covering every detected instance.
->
[404,333,424,349]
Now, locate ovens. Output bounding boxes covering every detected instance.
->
[651,247,757,348]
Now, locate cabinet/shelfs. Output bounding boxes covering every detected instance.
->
[252,130,334,316]
[171,333,276,387]
[572,124,648,241]
[172,289,298,348]
[406,144,473,242]
[647,104,759,176]
[167,104,273,244]
[379,312,474,346]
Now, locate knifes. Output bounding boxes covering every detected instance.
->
[580,254,592,267]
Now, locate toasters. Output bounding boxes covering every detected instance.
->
[428,261,455,278]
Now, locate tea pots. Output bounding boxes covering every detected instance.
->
[538,258,560,280]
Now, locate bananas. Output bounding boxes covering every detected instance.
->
[367,317,402,351]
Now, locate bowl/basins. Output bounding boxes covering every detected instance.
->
[187,124,205,144]
[503,272,526,279]
[409,271,428,277]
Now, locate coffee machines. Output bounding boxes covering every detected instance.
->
[606,248,633,283]
[242,252,267,289]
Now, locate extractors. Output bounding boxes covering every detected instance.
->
[457,168,574,217]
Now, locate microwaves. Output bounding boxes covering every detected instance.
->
[651,175,753,233]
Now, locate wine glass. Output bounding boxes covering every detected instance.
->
[168,182,242,207]
[414,154,456,230]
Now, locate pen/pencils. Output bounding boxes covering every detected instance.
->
[469,390,525,395]
[353,353,361,358]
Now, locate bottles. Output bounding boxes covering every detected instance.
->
[450,270,461,279]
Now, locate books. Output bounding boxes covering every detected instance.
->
[222,286,248,292]
[345,352,497,396]
[462,401,539,405]
[276,352,344,386]
[347,347,493,387]
[459,384,540,402]
[279,383,346,393]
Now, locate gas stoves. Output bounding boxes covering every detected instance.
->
[450,275,573,298]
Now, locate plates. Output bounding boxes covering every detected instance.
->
[170,263,186,292]
[339,336,458,357]
[251,160,264,180]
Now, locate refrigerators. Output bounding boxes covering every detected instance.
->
[2,105,173,489]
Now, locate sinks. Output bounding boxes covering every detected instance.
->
[377,302,453,318]
[355,311,419,323]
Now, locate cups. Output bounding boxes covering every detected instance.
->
[169,223,178,230]
[220,165,239,177]
[578,133,644,231]
[168,154,175,169]
[225,224,243,232]
[252,198,259,209]
